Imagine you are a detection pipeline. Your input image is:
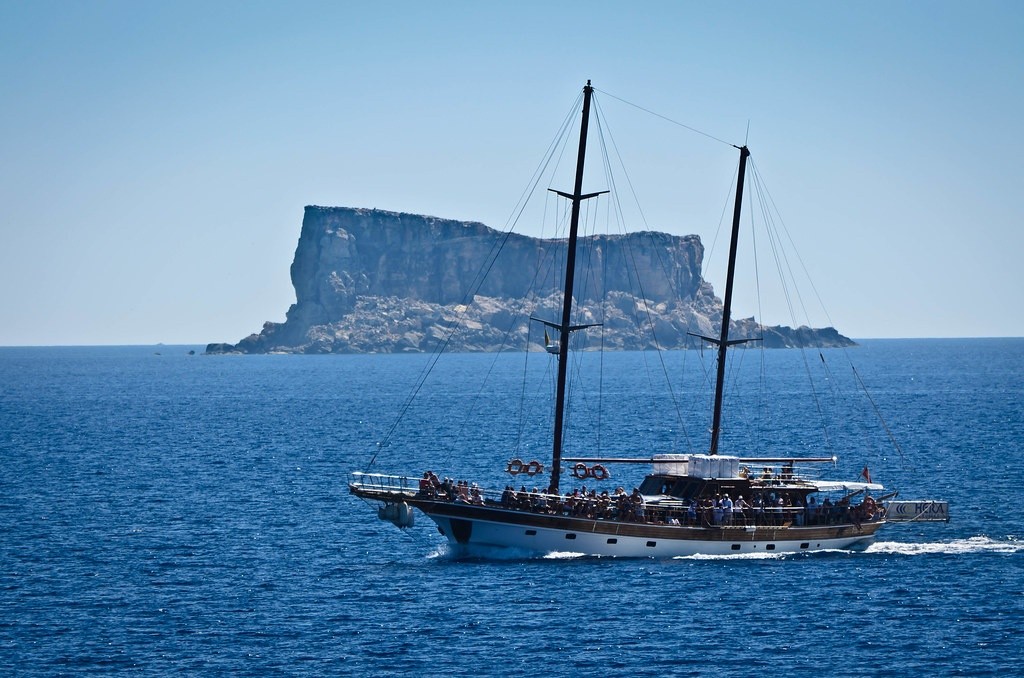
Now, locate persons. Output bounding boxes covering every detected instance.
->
[807,495,885,525]
[743,465,793,486]
[419,470,485,507]
[562,485,663,525]
[501,486,561,515]
[666,494,750,525]
[754,492,804,527]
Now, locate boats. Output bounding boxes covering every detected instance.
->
[348,78,951,556]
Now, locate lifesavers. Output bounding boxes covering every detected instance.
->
[526,461,539,477]
[574,462,588,479]
[865,497,875,509]
[592,465,606,479]
[510,458,522,474]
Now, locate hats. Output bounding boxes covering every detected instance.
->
[449,478,454,481]
[739,495,743,499]
[633,486,639,491]
[725,493,728,497]
[582,485,587,489]
[444,476,449,481]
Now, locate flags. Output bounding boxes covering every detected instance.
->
[862,468,872,483]
